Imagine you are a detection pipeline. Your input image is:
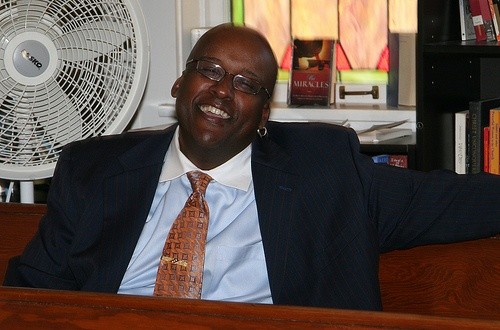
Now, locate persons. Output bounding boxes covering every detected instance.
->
[3,22,500,312]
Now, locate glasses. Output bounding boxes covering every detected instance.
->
[186,58,271,98]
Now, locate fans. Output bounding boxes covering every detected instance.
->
[0,0,149,204]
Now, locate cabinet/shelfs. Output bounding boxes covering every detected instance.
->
[414,0,499,176]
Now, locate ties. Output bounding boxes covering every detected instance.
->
[153,170,215,300]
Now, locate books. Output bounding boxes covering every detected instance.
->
[287,39,337,106]
[356,119,414,141]
[459,0,500,42]
[456,97,500,175]
